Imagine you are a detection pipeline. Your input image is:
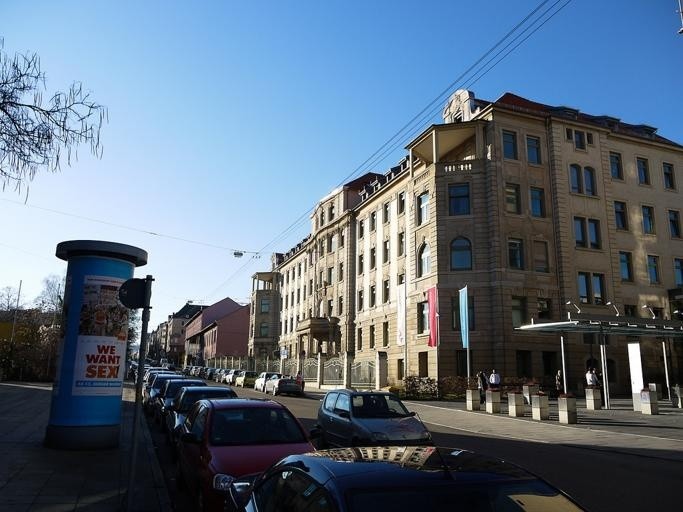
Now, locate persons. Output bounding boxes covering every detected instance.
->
[488,368,501,388]
[555,369,564,399]
[592,368,603,388]
[477,371,490,405]
[598,371,605,407]
[585,367,596,388]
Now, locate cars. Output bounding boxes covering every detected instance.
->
[228,445,591,512]
[316,389,435,447]
[189,366,302,396]
[143,367,316,491]
